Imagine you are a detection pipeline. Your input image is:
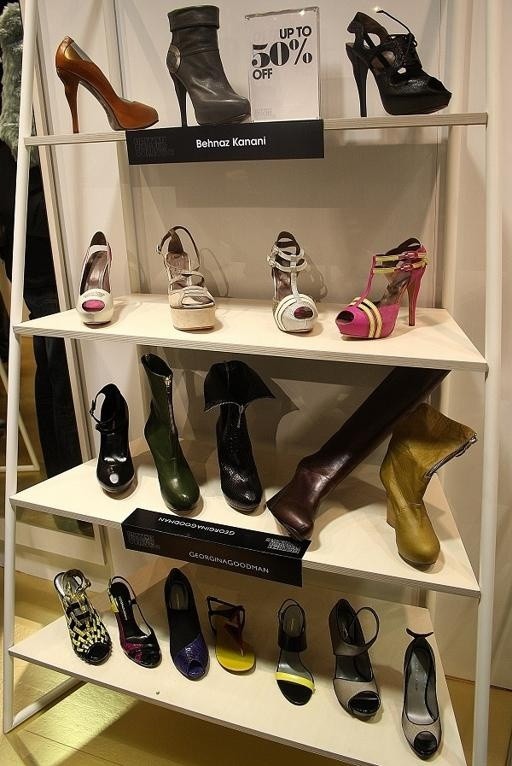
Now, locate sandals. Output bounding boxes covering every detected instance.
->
[156,227,216,330]
[206,595,256,672]
[269,232,318,333]
[77,231,114,325]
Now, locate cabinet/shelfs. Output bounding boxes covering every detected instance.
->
[0,5,509,766]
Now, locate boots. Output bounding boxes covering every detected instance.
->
[204,360,276,515]
[166,6,250,127]
[264,366,452,542]
[379,401,474,561]
[141,354,201,515]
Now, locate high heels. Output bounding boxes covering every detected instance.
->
[336,239,427,339]
[274,597,314,706]
[346,10,451,116]
[402,628,442,759]
[53,569,113,666]
[106,575,161,668]
[328,598,381,717]
[164,568,209,679]
[56,36,158,134]
[90,383,135,493]
